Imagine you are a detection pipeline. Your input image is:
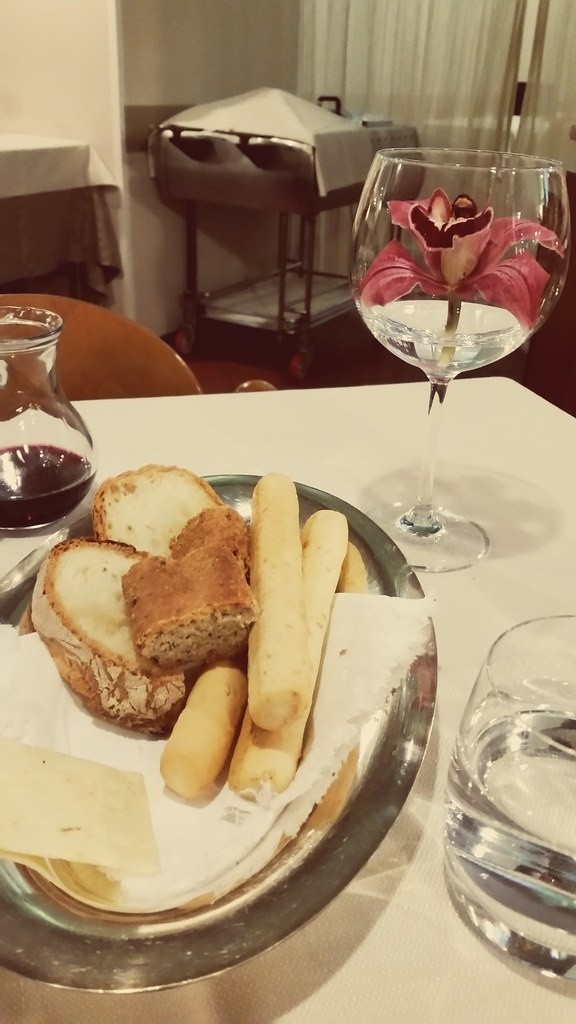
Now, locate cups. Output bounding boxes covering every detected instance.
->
[439,614,576,980]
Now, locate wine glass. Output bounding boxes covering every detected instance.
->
[346,148,571,574]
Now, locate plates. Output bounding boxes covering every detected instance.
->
[0,475,437,995]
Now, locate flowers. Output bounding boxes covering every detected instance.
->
[358,187,567,367]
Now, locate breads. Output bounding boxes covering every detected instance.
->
[161,657,247,795]
[121,545,259,663]
[170,509,248,567]
[229,511,349,794]
[30,537,186,734]
[250,474,307,729]
[91,464,225,559]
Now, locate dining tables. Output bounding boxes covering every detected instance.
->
[0,377,576,1023]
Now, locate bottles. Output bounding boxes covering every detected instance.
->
[0,305,98,531]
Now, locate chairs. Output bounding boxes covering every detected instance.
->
[0,291,202,414]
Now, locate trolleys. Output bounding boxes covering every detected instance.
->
[153,95,424,387]
